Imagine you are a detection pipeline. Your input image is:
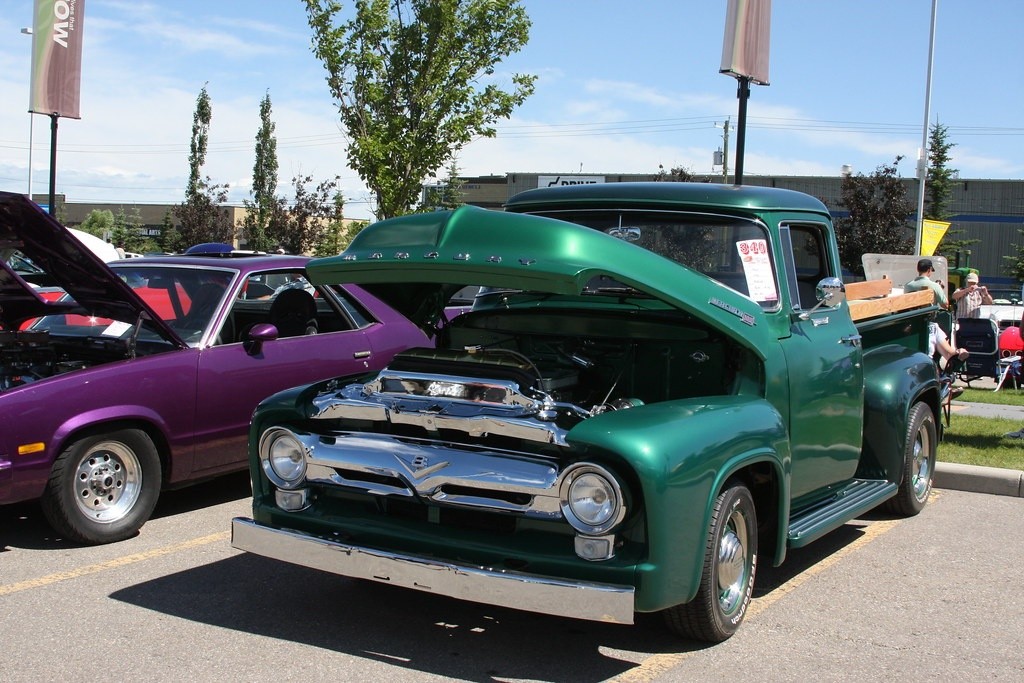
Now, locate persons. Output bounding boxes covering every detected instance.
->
[952,272,993,380]
[928,310,969,405]
[903,259,949,309]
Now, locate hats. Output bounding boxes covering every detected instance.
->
[965,272,978,288]
[917,259,936,272]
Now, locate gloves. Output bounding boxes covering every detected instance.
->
[955,348,969,361]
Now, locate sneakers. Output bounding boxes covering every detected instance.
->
[1002,427,1024,440]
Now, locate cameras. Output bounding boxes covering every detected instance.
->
[975,287,982,291]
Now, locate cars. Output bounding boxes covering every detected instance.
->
[0,189,472,548]
[230,180,946,643]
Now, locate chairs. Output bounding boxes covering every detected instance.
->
[952,317,1021,392]
